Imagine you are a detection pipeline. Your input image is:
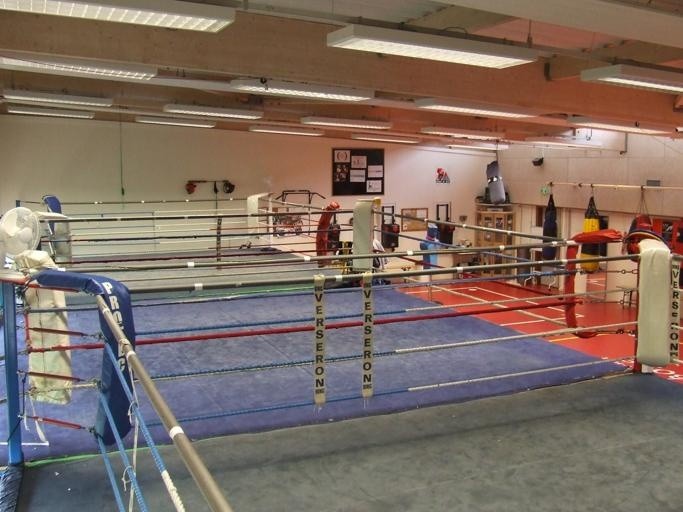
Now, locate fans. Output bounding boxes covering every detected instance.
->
[0,207,41,261]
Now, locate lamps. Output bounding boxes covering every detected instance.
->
[248,122,327,139]
[299,115,394,131]
[412,95,541,120]
[2,1,239,37]
[226,75,377,104]
[532,148,545,167]
[350,131,421,147]
[1,48,157,84]
[134,115,219,130]
[419,124,508,141]
[525,134,605,149]
[578,62,683,97]
[162,102,266,122]
[1,87,115,110]
[445,142,500,151]
[324,22,540,72]
[564,113,672,139]
[7,104,98,121]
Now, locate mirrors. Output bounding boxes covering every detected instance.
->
[585,215,609,301]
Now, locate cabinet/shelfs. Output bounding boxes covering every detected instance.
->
[474,203,517,281]
